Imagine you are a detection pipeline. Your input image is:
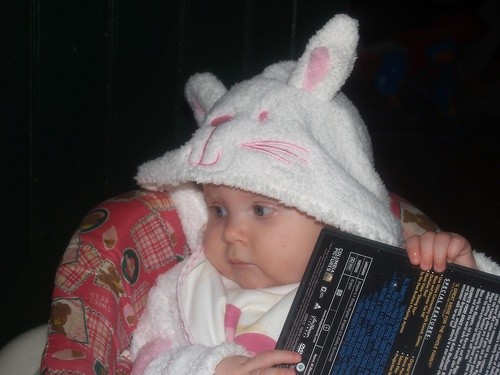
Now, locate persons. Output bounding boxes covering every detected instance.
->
[128,12,500,375]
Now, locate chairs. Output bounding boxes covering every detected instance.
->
[40,190,444,375]
